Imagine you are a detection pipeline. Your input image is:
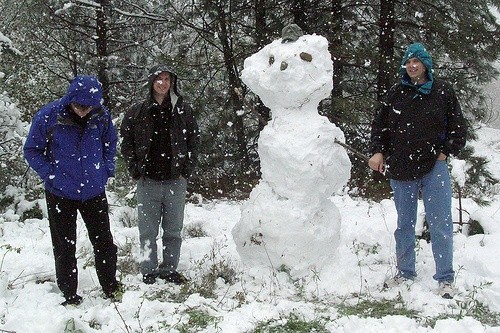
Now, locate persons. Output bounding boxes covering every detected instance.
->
[23,75,120,307]
[120,65,201,285]
[367,43,467,299]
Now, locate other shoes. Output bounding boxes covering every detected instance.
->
[143,271,158,285]
[160,270,187,284]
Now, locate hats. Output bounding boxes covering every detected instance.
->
[280,23,303,42]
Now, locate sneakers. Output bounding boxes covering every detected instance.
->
[439,281,456,298]
[383,274,414,291]
